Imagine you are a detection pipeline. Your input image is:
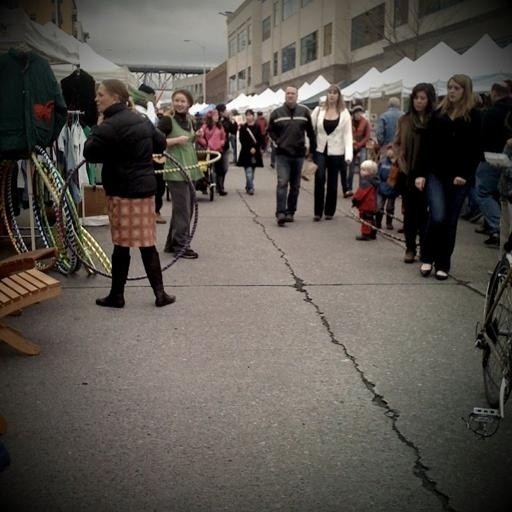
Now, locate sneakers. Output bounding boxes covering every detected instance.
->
[314,215,331,221]
[156,213,167,224]
[203,186,227,196]
[245,186,254,194]
[355,223,404,240]
[277,212,293,226]
[164,243,198,259]
[344,190,353,199]
[404,249,417,263]
[462,209,499,248]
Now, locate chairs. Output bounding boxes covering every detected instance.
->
[0,268,61,355]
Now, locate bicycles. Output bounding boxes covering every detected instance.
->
[464,221,510,458]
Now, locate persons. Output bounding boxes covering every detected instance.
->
[83,80,175,308]
[346,98,405,240]
[391,74,511,279]
[268,83,353,226]
[239,110,268,194]
[135,84,239,259]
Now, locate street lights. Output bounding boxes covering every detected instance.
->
[184,36,209,106]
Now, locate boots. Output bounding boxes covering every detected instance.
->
[141,252,175,307]
[96,254,131,308]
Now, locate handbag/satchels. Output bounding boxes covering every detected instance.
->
[302,159,318,181]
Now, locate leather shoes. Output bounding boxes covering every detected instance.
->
[419,264,448,280]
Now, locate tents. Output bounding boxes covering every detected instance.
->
[224,33,512,118]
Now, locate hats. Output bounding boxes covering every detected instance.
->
[205,109,218,117]
[351,104,363,113]
